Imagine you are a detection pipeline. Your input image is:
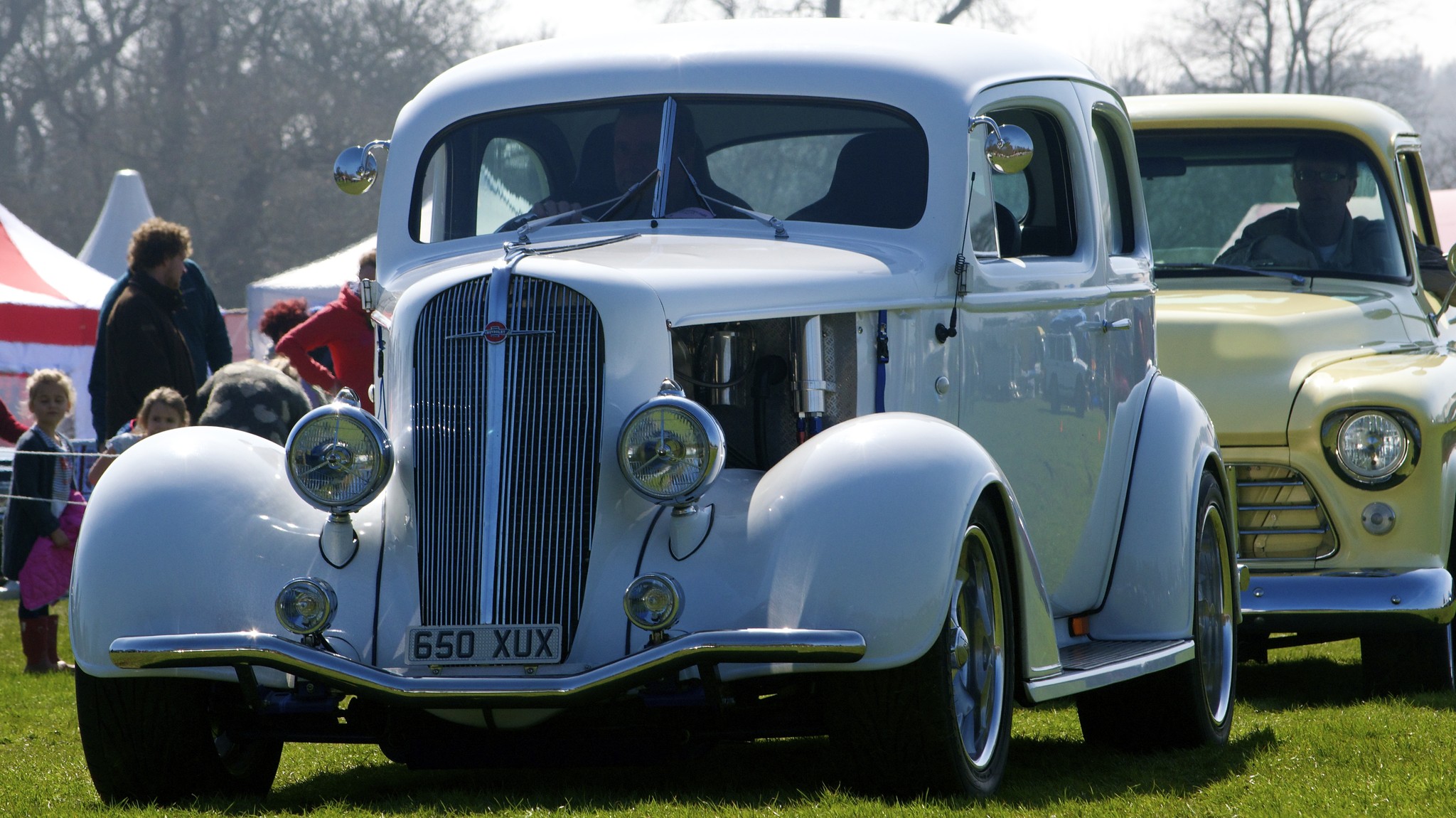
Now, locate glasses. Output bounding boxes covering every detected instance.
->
[1292,169,1355,184]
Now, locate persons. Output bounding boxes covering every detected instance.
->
[85,259,231,454]
[87,386,192,487]
[194,355,313,448]
[0,399,31,591]
[0,367,82,675]
[526,94,723,221]
[102,218,197,430]
[277,253,378,415]
[1208,129,1445,280]
[257,299,326,407]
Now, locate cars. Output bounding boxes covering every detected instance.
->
[71,12,1241,805]
[1120,92,1455,693]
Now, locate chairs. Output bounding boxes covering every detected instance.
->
[783,129,1024,257]
